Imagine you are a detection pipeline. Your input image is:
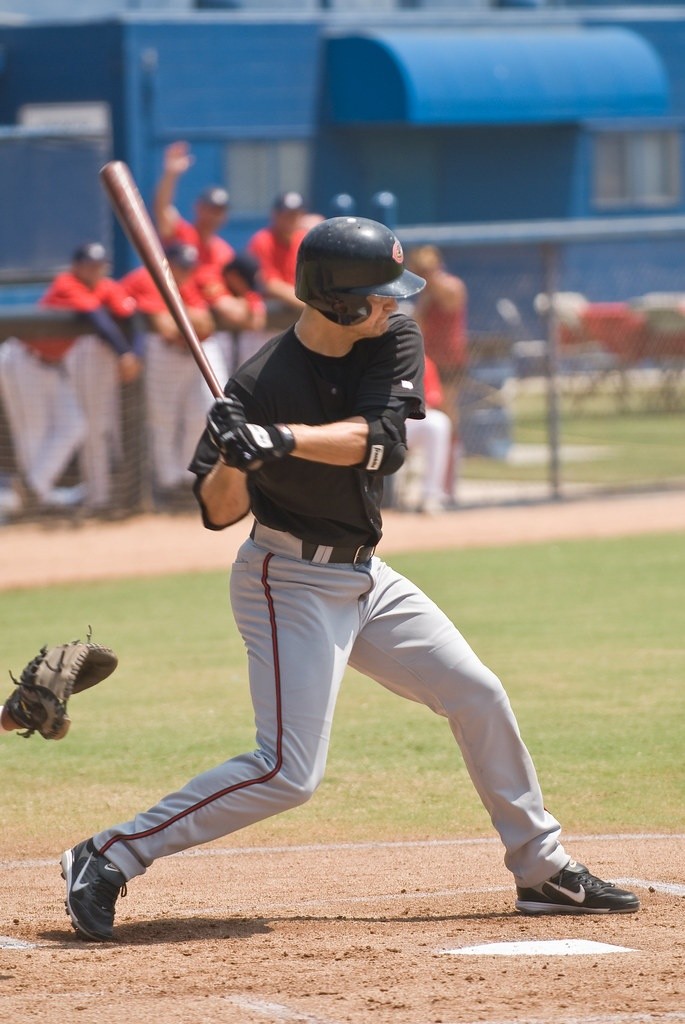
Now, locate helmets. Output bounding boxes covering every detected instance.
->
[294,216,427,327]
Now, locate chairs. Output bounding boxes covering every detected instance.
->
[547,289,685,420]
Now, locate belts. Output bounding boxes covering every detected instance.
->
[250,519,376,568]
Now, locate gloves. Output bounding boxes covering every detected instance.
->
[219,422,297,472]
[206,393,247,451]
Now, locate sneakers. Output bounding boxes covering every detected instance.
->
[59,836,127,942]
[515,859,640,913]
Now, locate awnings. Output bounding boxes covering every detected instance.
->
[326,29,674,130]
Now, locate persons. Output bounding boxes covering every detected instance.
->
[1,142,466,521]
[0,641,118,740]
[59,217,639,943]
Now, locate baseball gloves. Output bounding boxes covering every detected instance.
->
[5,625,119,741]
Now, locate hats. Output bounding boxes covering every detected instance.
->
[167,243,199,269]
[234,255,260,292]
[206,187,228,210]
[274,190,302,212]
[74,242,114,266]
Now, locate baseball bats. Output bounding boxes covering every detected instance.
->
[99,159,264,471]
